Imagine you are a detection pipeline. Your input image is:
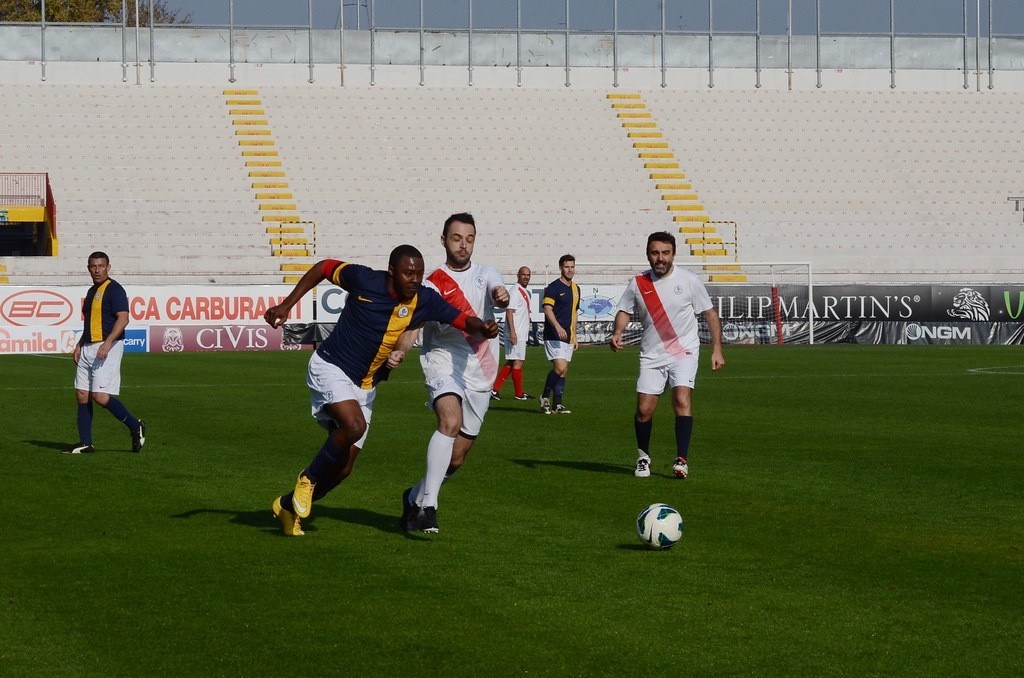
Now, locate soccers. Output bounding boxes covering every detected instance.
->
[636,502,684,549]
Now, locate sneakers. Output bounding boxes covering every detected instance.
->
[551,404,571,414]
[539,395,551,414]
[272,496,305,536]
[131,420,145,453]
[491,389,500,401]
[61,443,95,454]
[515,393,536,400]
[634,455,651,477]
[403,487,421,531]
[292,469,316,518]
[421,506,440,534]
[673,456,688,479]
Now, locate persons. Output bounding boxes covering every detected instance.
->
[539,254,581,414]
[609,232,725,479]
[60,252,146,454]
[387,211,510,534]
[263,245,499,536]
[491,267,532,400]
